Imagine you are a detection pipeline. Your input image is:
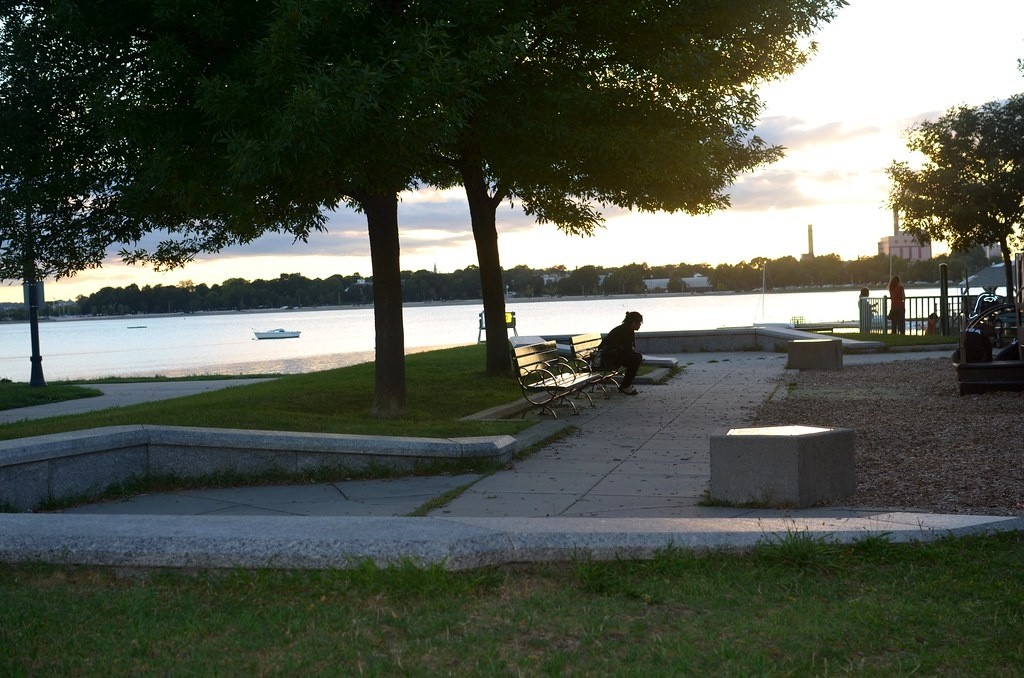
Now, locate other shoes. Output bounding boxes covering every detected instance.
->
[620,384,638,395]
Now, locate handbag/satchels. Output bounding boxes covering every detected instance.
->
[590,347,606,370]
[888,310,892,320]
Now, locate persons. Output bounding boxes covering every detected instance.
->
[858,287,879,334]
[889,275,906,335]
[597,309,644,396]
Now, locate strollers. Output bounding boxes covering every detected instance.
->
[966,294,1005,348]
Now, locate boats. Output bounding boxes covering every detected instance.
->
[127,325,148,328]
[253,328,301,339]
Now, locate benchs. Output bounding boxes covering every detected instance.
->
[510,339,602,420]
[994,312,1024,348]
[567,332,628,400]
